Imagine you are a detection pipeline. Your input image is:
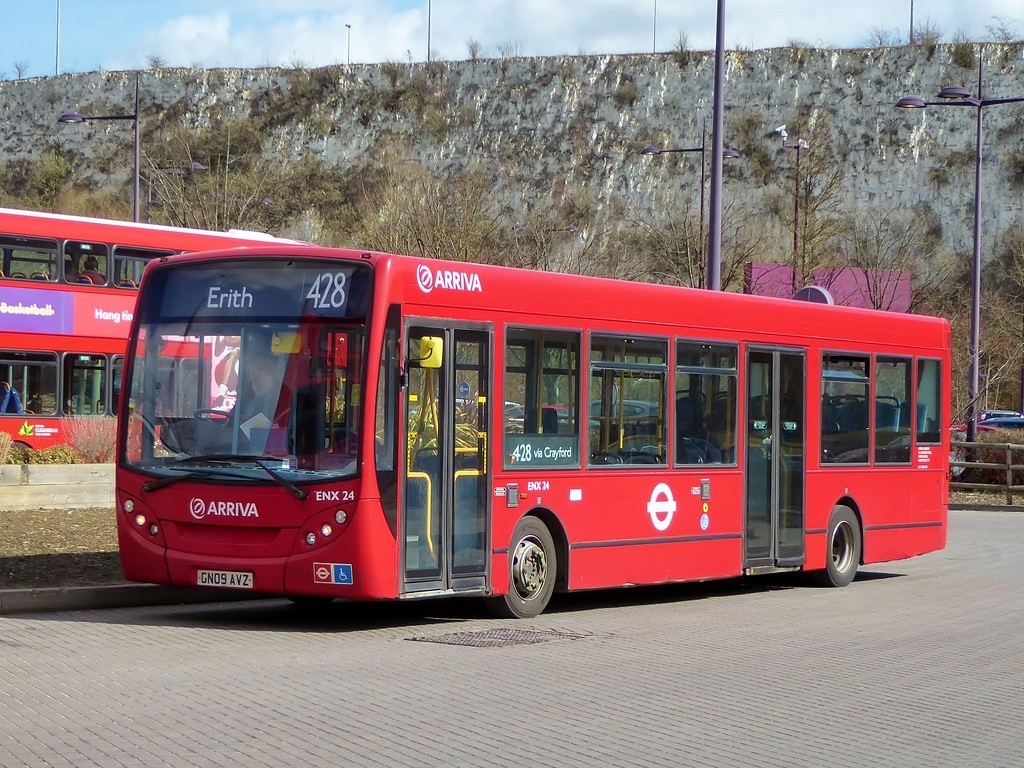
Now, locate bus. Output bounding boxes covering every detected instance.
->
[0,206,322,464]
[109,242,954,621]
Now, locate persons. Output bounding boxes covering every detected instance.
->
[55,256,105,285]
[206,352,292,456]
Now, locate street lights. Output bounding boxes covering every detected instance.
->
[143,153,206,223]
[55,74,145,289]
[893,53,1024,496]
[344,23,352,65]
[639,116,740,290]
[165,167,191,229]
[767,124,809,297]
[407,49,412,63]
[230,193,276,230]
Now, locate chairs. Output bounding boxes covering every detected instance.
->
[30,272,49,281]
[71,275,93,284]
[0,382,24,414]
[116,279,136,288]
[527,408,558,433]
[412,447,478,501]
[676,389,929,435]
[11,273,26,279]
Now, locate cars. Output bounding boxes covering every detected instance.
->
[409,396,660,426]
[949,407,1024,463]
